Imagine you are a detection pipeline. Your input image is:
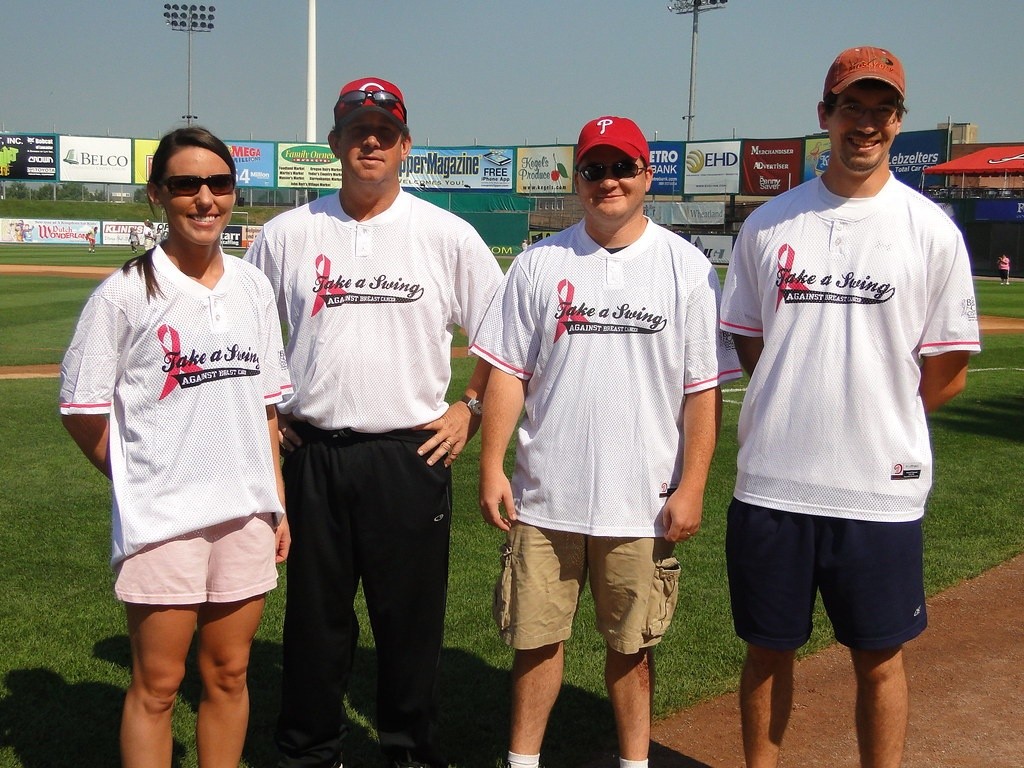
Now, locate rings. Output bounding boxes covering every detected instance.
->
[686,531,691,536]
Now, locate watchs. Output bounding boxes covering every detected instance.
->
[460,394,484,416]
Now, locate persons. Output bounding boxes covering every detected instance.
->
[242,77,506,768]
[997,253,1010,285]
[59,128,293,768]
[469,116,745,768]
[129,219,161,254]
[86,227,98,254]
[522,240,531,251]
[721,46,981,768]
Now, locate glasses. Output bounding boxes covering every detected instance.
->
[334,90,407,127]
[158,174,236,195]
[830,103,898,121]
[576,160,647,181]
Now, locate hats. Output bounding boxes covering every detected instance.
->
[576,115,650,167]
[334,77,405,130]
[823,46,906,101]
